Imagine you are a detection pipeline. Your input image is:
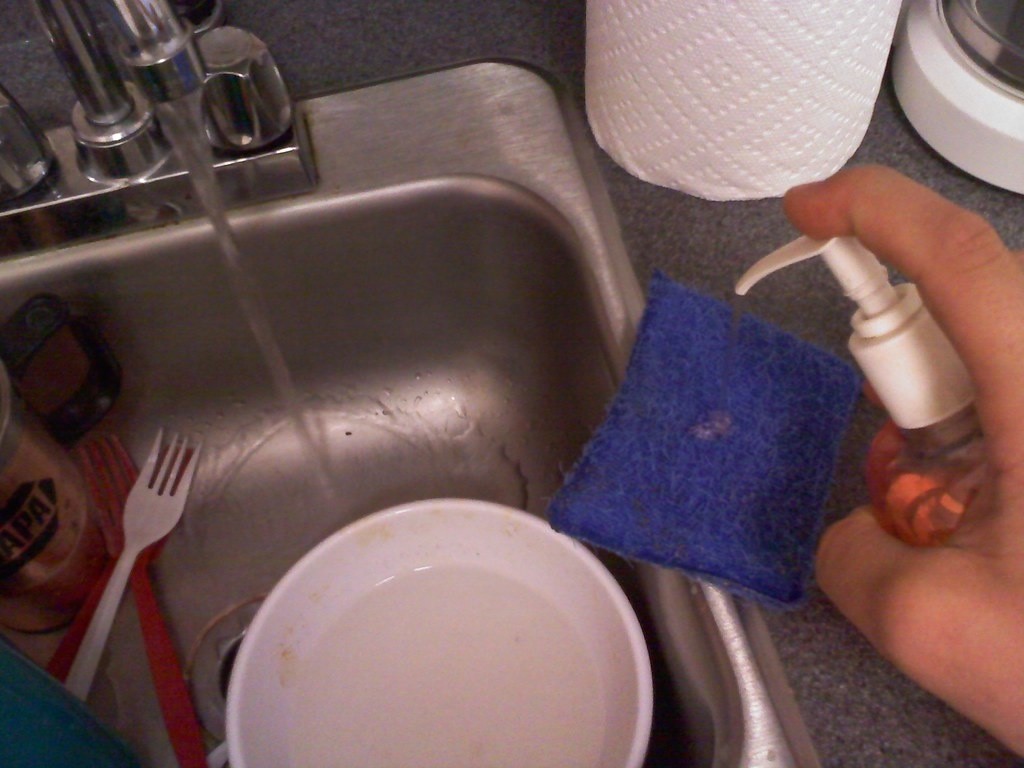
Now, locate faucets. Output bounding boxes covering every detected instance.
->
[24,0,207,179]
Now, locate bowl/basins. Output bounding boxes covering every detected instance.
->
[222,504,651,768]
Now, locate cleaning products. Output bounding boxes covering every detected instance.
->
[732,226,1004,554]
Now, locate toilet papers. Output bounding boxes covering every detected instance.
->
[581,0,908,207]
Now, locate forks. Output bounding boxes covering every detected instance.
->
[78,434,214,768]
[65,425,202,702]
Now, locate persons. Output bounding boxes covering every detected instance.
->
[785,163,1023,759]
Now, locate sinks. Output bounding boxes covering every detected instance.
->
[0,57,831,767]
